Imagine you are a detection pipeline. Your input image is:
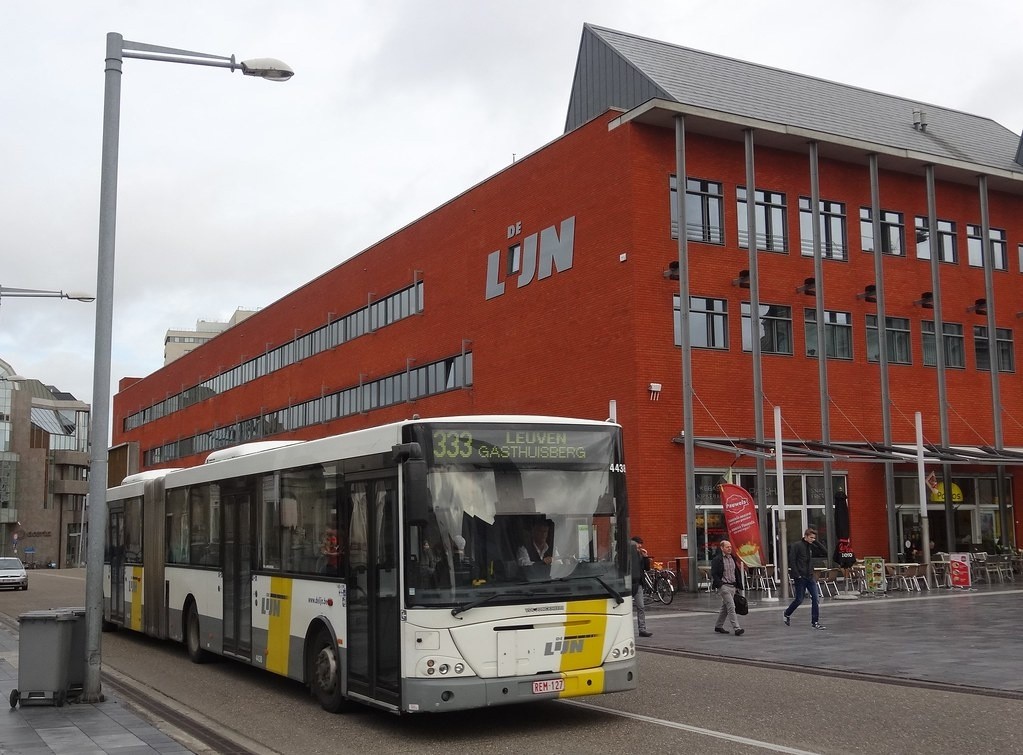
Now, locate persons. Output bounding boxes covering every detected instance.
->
[515,521,575,566]
[929,540,941,566]
[783,528,826,630]
[905,542,917,563]
[711,540,744,635]
[614,537,652,637]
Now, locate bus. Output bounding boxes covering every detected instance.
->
[77,413,640,718]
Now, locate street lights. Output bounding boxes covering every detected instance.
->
[85,33,298,708]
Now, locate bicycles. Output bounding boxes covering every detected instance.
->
[642,562,679,605]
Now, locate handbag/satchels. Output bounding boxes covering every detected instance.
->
[734,591,748,615]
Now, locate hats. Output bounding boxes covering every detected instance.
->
[631,537,643,543]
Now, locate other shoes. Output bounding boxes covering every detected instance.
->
[735,629,745,636]
[715,627,729,634]
[639,630,653,637]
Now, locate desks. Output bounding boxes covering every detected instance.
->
[787,567,827,595]
[748,565,775,589]
[931,560,950,587]
[698,566,714,592]
[883,563,918,591]
[851,565,865,593]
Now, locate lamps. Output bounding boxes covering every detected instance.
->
[648,381,662,401]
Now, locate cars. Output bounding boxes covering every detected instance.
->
[0,557,28,590]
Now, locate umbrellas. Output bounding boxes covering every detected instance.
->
[832,487,857,591]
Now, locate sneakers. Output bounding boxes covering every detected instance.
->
[812,624,826,629]
[783,611,791,626]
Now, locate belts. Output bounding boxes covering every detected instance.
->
[723,582,735,586]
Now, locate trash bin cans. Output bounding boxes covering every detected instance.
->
[49,607,102,693]
[9,610,80,708]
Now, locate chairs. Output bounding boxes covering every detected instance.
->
[698,551,1023,592]
